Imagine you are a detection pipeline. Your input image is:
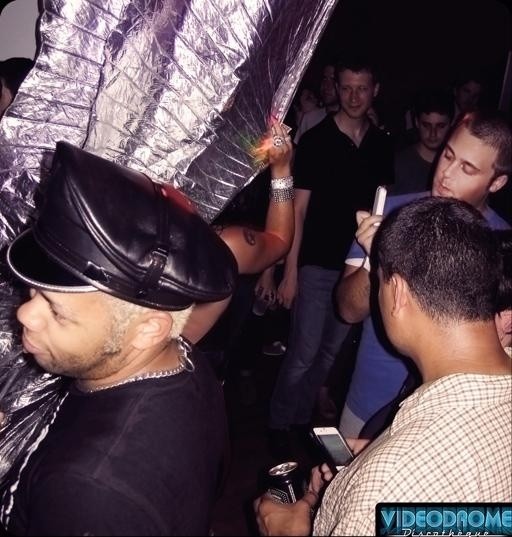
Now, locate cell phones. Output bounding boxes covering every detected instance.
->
[371,186,388,227]
[309,426,355,474]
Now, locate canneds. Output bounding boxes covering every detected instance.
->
[267,462,304,504]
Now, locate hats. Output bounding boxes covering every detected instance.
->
[6,142,241,313]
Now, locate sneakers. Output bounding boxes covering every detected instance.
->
[318,387,337,420]
[260,338,285,356]
